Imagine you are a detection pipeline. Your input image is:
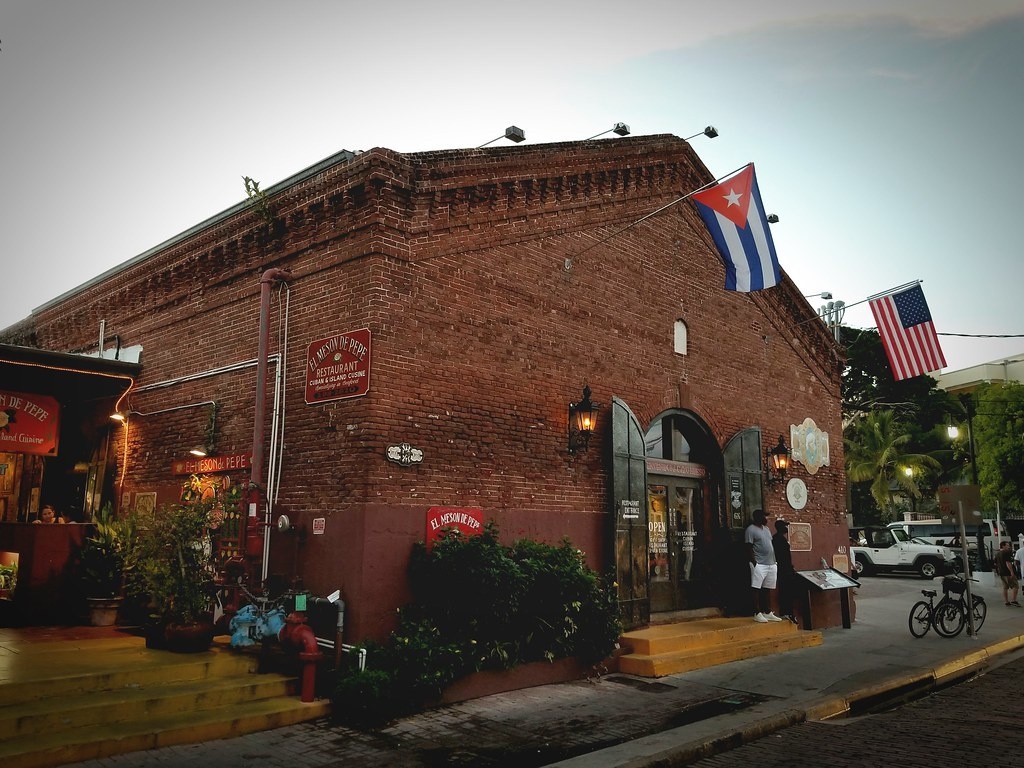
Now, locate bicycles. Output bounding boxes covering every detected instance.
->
[931,576,987,638]
[909,569,962,639]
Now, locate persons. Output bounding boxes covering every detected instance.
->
[771,519,799,626]
[745,510,782,623]
[677,510,688,579]
[31,505,65,524]
[690,164,781,292]
[996,541,1022,607]
[1014,542,1024,598]
[849,537,859,596]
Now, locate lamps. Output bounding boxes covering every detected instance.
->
[684,126,719,141]
[586,122,630,140]
[109,410,130,421]
[568,385,601,454]
[189,444,207,457]
[476,125,525,148]
[767,434,793,485]
[766,213,779,223]
[805,292,832,299]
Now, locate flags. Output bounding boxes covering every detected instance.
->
[869,284,948,382]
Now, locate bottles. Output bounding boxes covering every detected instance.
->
[820,556,829,570]
[963,605,967,614]
[58,515,65,523]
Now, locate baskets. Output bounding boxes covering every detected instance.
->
[944,578,966,594]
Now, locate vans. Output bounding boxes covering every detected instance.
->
[858,519,1012,568]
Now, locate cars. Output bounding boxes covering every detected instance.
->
[848,525,952,581]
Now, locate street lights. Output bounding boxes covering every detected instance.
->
[904,463,918,521]
[948,411,992,573]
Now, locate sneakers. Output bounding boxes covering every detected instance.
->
[785,612,799,625]
[763,611,782,622]
[1005,602,1011,606]
[754,612,768,623]
[1010,601,1021,607]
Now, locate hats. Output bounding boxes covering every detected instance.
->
[752,510,770,518]
[775,520,790,527]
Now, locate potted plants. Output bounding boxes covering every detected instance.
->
[79,487,238,653]
